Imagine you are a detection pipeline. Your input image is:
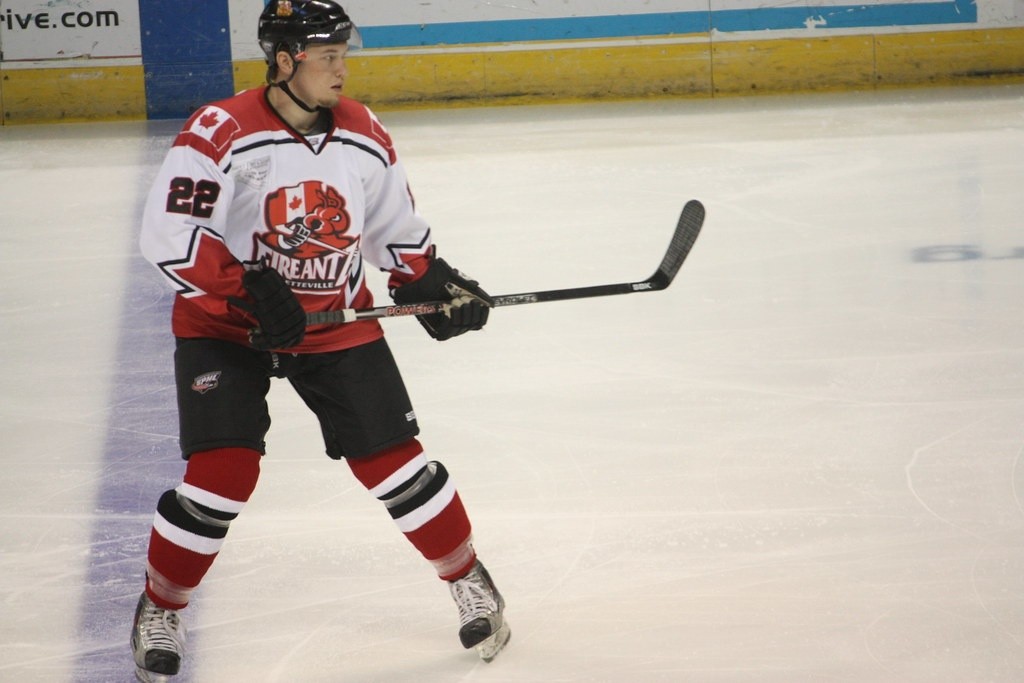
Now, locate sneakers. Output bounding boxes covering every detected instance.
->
[129,591,187,683]
[448,558,510,662]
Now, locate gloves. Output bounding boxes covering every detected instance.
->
[227,267,307,350]
[389,244,494,342]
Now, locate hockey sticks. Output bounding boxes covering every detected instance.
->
[225,200,705,331]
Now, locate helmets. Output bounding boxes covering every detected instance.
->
[258,0,351,63]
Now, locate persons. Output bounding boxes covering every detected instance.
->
[131,0,513,683]
